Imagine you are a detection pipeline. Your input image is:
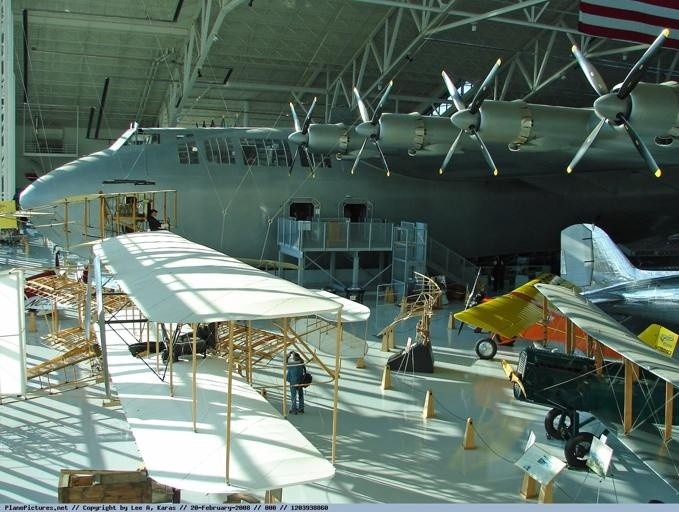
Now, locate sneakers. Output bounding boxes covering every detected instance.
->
[289,408,304,414]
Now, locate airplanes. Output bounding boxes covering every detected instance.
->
[21,28,679,271]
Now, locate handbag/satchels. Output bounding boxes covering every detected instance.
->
[302,373,312,387]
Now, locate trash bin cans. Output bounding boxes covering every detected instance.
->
[344,287,365,304]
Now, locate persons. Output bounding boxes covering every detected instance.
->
[286,350,304,415]
[147,209,169,231]
